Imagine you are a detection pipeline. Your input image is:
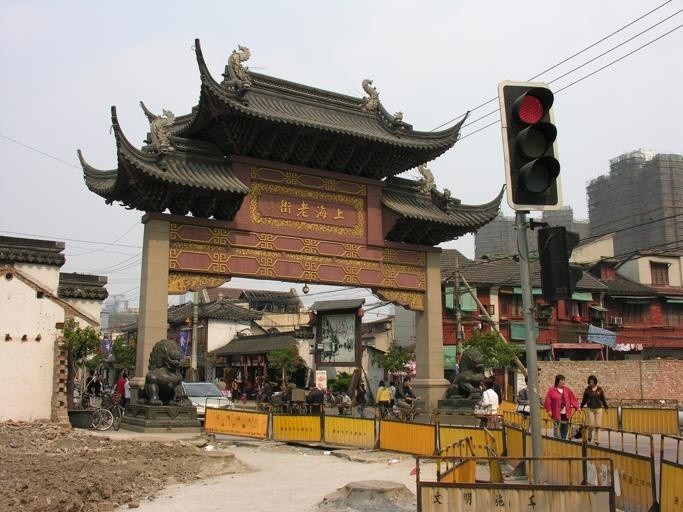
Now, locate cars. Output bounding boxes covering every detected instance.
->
[176,382,237,421]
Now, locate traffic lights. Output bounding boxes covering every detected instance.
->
[501,84,561,209]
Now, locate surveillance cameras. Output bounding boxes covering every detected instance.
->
[303,287,310,295]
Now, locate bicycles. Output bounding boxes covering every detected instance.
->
[76,387,125,432]
[384,398,432,422]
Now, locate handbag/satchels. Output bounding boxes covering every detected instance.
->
[575,425,588,439]
[473,404,497,415]
[515,404,530,413]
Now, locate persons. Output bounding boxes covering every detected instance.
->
[517,375,544,415]
[356,383,368,418]
[376,376,421,421]
[477,375,501,427]
[117,371,132,415]
[84,368,105,397]
[338,390,353,417]
[544,372,582,439]
[580,375,609,444]
[216,375,335,414]
